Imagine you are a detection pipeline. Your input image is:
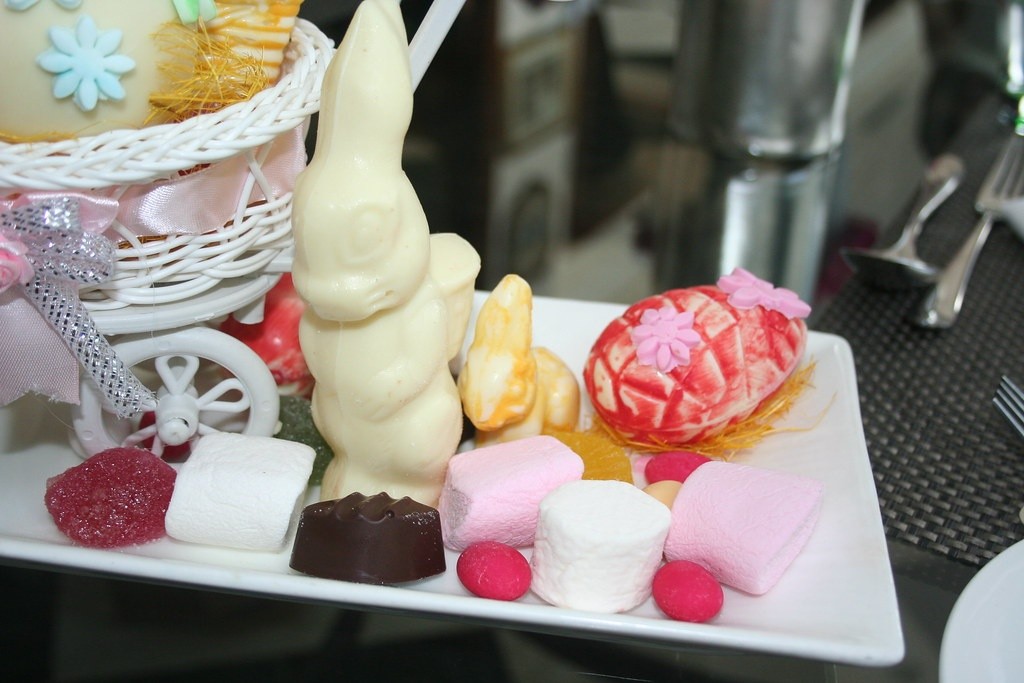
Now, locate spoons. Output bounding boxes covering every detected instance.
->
[839,153,966,291]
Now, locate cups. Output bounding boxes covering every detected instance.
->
[661,0,865,160]
[648,157,836,309]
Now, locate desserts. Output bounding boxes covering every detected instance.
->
[0,1,820,627]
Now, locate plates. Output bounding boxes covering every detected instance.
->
[939,539,1024,683]
[1,291,906,667]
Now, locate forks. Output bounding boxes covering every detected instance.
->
[909,135,1024,329]
[992,375,1024,439]
